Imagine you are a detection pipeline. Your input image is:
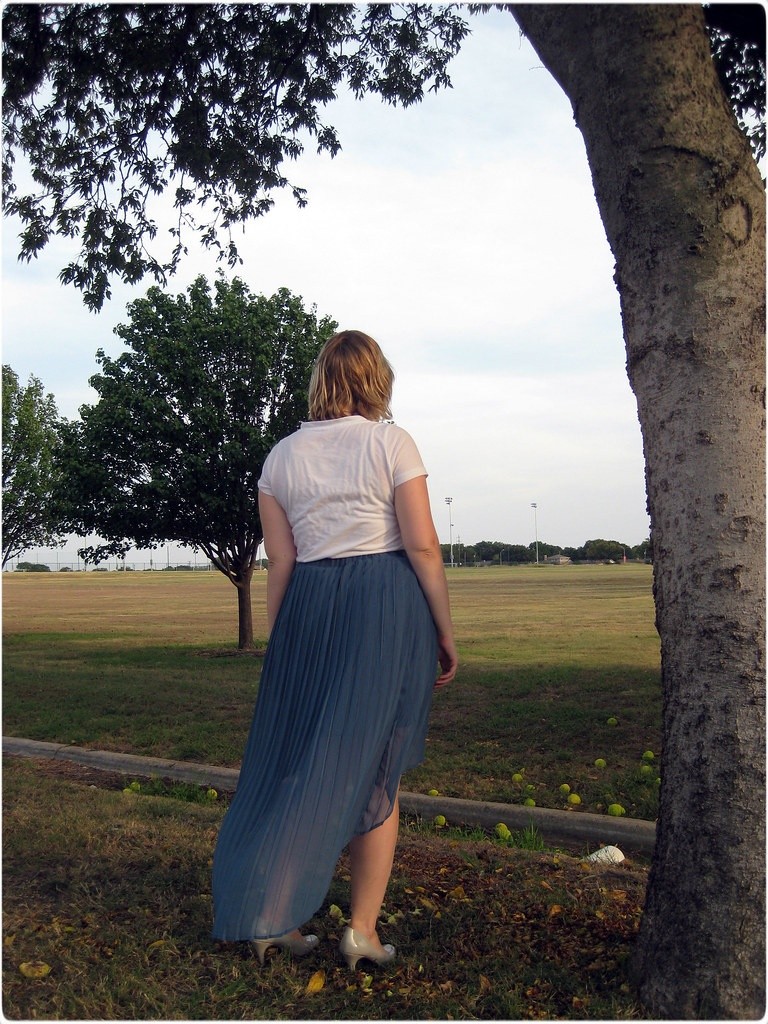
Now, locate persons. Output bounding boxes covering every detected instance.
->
[213,330,457,971]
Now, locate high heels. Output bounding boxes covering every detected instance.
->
[250,921,320,965]
[340,927,396,972]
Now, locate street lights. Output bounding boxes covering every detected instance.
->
[444,496,453,567]
[499,548,505,568]
[530,502,538,565]
